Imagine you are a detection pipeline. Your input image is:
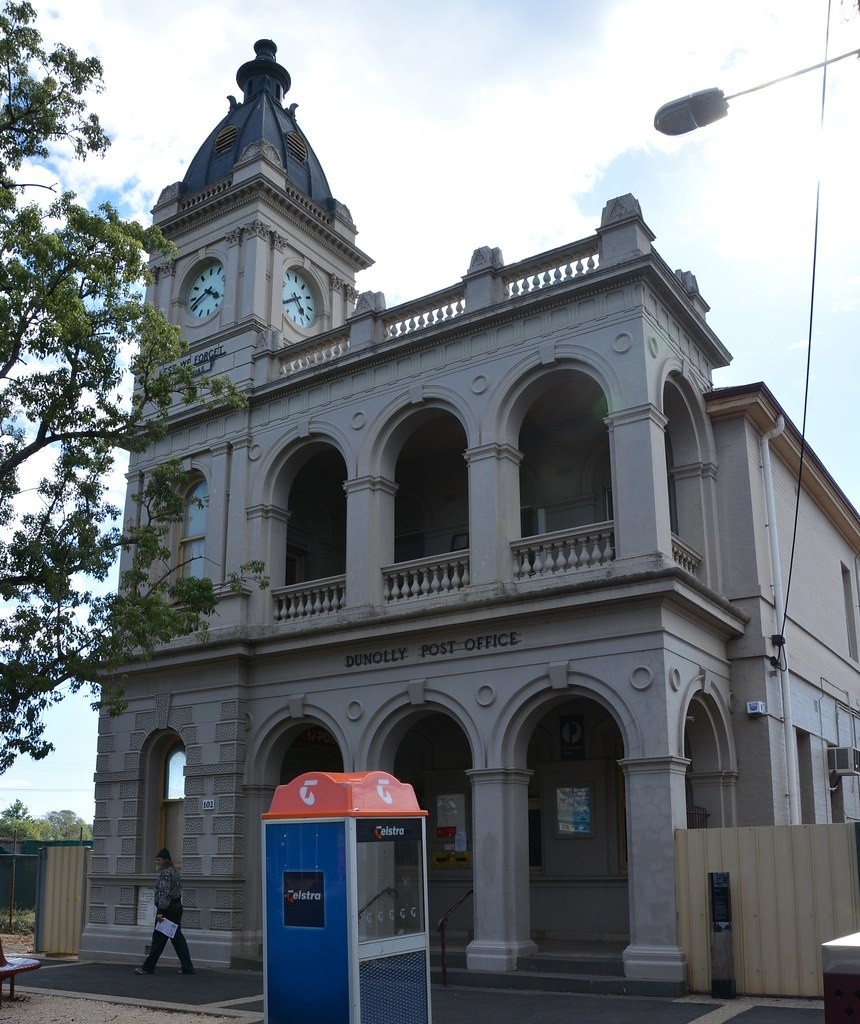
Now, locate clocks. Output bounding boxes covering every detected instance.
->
[283,271,316,329]
[187,263,224,316]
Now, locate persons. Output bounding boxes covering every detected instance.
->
[133,848,196,976]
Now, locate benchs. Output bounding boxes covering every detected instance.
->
[0,957,41,997]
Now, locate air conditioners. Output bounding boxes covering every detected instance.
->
[828,746,860,793]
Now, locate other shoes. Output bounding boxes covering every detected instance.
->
[133,968,152,974]
[177,970,195,975]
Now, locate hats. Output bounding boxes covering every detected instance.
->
[155,848,171,859]
